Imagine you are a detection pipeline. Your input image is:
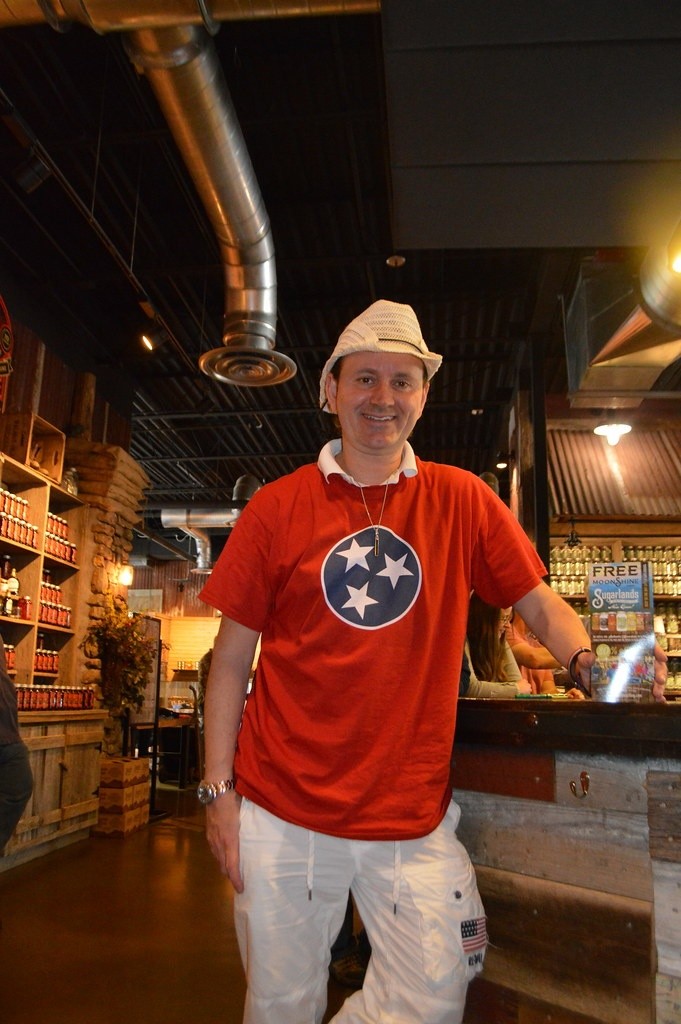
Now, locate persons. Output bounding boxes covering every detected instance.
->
[464,590,532,699]
[505,606,587,700]
[198,635,220,716]
[0,637,34,852]
[205,299,668,1024]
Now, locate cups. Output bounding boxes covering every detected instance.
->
[178,661,200,670]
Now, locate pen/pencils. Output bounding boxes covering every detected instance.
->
[514,692,555,700]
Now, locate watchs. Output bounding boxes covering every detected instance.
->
[198,780,236,804]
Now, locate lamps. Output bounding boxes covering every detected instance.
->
[494,450,508,469]
[563,519,581,546]
[141,328,168,350]
[593,417,632,445]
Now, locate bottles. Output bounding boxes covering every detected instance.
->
[552,544,681,692]
[0,467,78,626]
[3,633,95,711]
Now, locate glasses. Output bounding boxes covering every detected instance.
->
[499,617,509,624]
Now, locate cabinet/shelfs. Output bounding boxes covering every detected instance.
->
[550,537,681,695]
[0,452,90,684]
[0,709,111,873]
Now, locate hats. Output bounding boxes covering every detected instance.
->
[319,298,443,413]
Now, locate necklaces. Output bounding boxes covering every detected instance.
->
[339,452,391,557]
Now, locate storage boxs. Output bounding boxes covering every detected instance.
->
[90,756,151,839]
[0,413,67,486]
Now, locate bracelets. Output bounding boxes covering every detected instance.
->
[568,646,592,682]
